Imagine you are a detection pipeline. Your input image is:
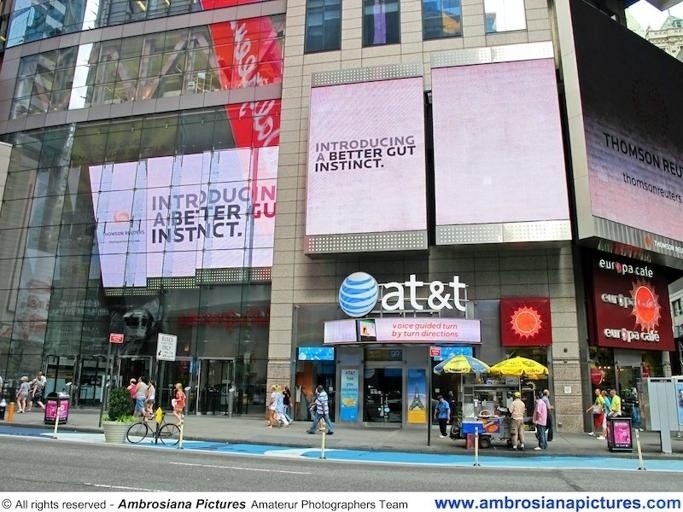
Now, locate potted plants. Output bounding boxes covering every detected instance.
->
[101,386,139,443]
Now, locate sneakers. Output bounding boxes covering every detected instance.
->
[587,432,607,441]
[306,427,334,436]
[439,434,448,439]
[512,432,547,451]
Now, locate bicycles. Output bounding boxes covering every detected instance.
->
[126,410,181,447]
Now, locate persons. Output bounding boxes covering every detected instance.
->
[533,390,547,450]
[15,371,47,413]
[266,384,334,435]
[433,395,450,439]
[173,383,187,423]
[542,389,555,441]
[585,388,622,440]
[127,374,156,420]
[509,391,525,450]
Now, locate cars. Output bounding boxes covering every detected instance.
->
[432,398,439,409]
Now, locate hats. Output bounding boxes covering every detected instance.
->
[513,391,520,398]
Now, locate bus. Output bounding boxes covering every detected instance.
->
[245,382,266,404]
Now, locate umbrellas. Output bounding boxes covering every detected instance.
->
[491,355,549,390]
[433,355,490,393]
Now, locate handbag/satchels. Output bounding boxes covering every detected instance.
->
[592,405,602,415]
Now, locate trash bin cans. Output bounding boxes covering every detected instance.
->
[606,411,635,452]
[44,392,70,424]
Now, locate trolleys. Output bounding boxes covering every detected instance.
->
[460,372,524,448]
